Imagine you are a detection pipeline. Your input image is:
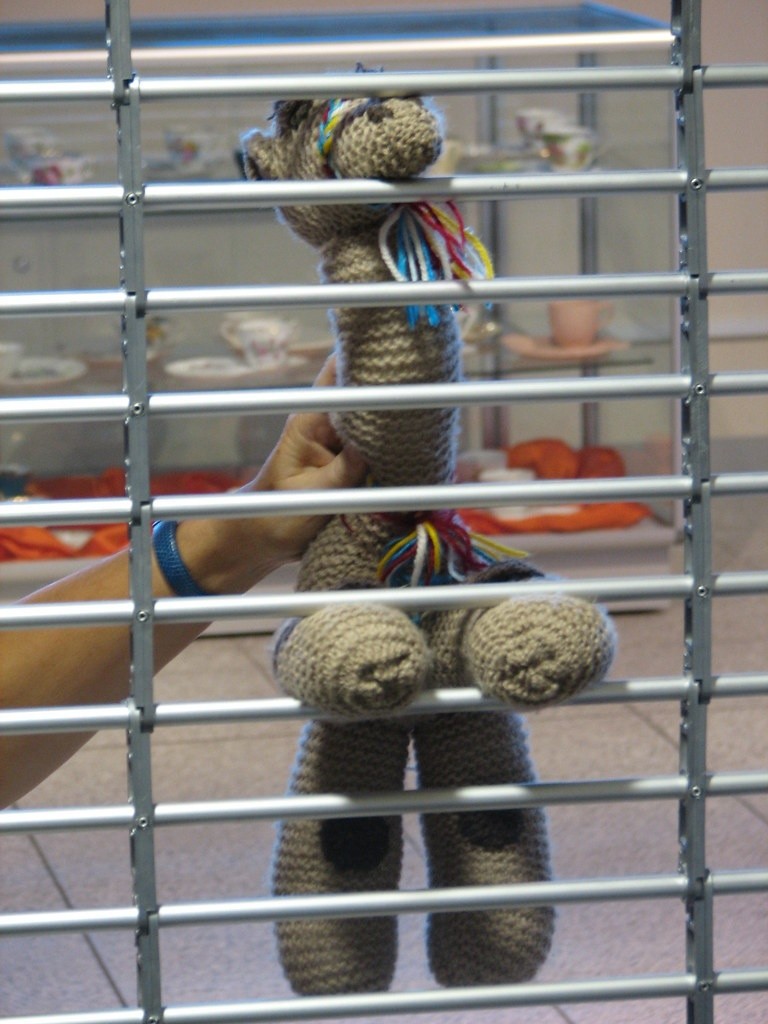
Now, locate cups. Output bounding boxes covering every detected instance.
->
[220,312,295,370]
[548,298,614,346]
[517,108,612,168]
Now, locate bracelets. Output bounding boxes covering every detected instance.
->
[149,517,240,600]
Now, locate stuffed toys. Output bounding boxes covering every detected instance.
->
[233,62,619,996]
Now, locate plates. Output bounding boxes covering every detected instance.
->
[0,357,88,386]
[165,357,244,379]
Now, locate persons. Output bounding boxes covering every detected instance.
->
[0,344,379,814]
